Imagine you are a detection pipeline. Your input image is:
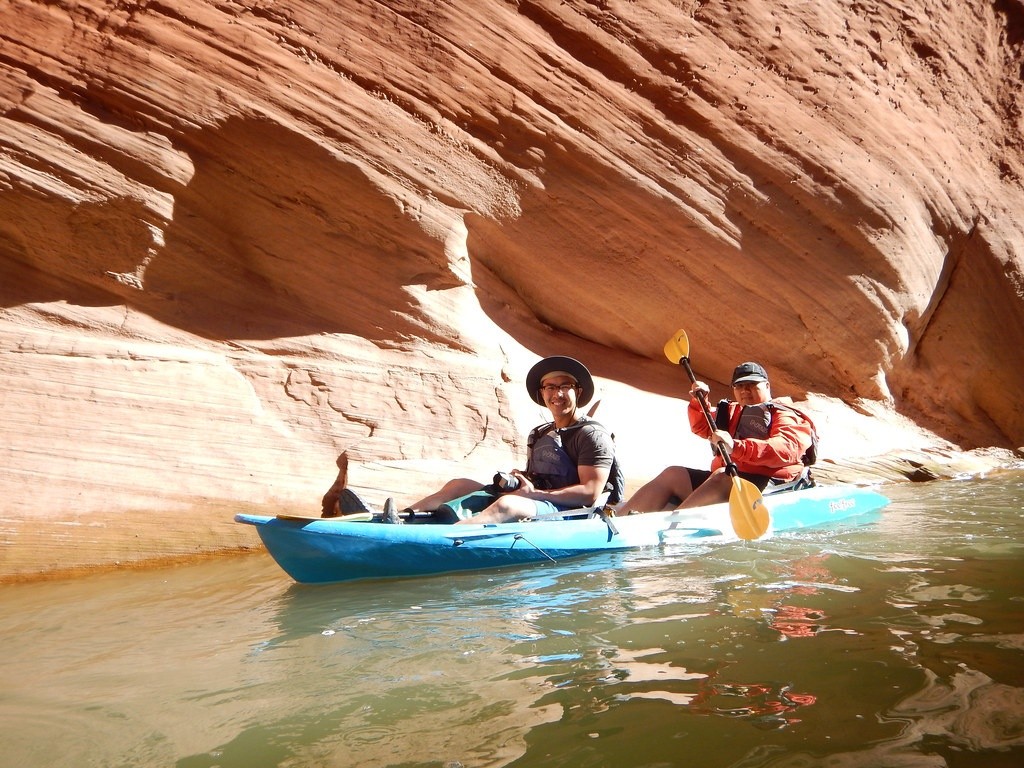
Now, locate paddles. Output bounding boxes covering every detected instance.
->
[277,510,437,520]
[663,329,770,540]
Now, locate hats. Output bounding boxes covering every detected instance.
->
[526,355,594,408]
[730,362,768,388]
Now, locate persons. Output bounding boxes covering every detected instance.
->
[338,356,625,525]
[604,362,817,517]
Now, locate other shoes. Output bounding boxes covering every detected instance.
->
[382,498,404,524]
[603,504,616,518]
[627,509,640,515]
[339,489,372,516]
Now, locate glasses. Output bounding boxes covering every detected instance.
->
[734,382,758,390]
[541,382,578,394]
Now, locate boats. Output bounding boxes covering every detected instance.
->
[234,482,891,583]
